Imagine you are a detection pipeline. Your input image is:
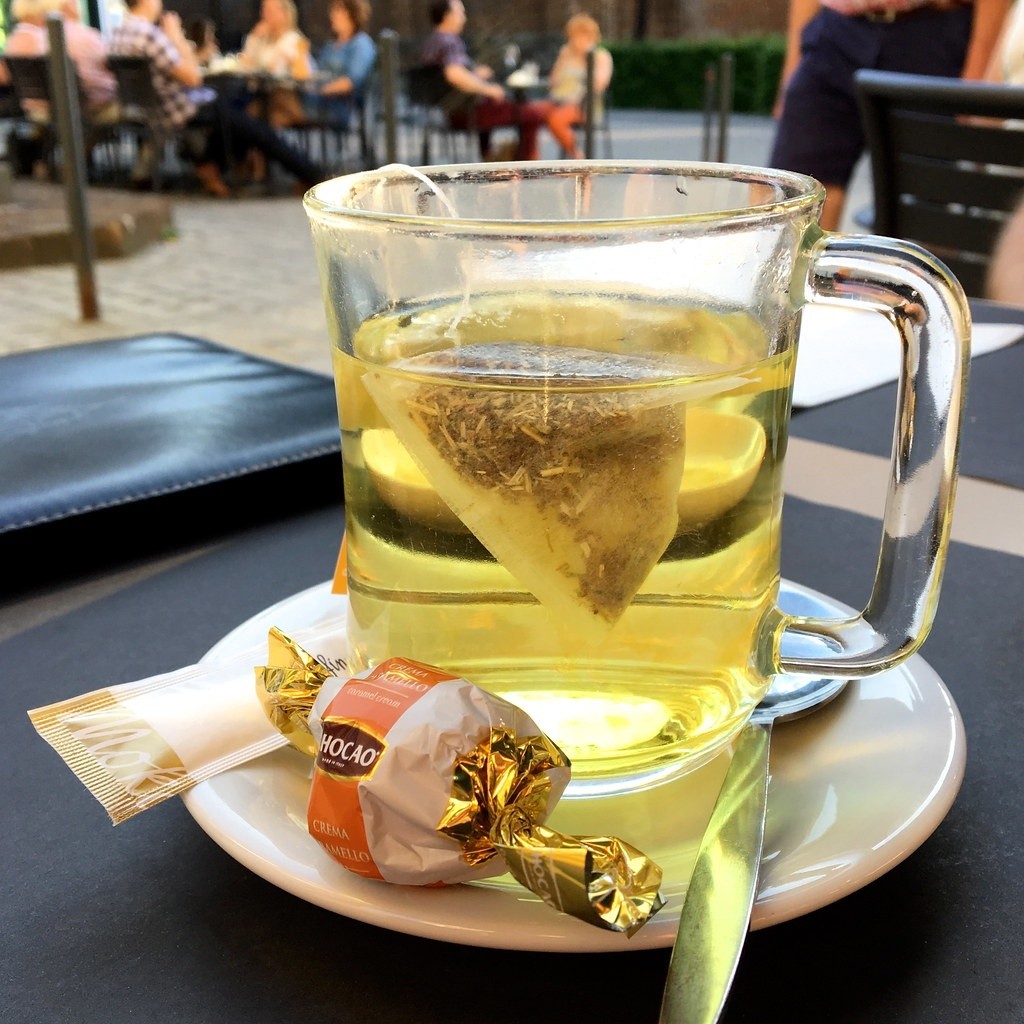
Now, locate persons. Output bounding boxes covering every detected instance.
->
[0,0,376,198]
[527,11,614,160]
[766,0,1023,306]
[413,0,543,162]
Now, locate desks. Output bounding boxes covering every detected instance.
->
[1,297,1024,1024]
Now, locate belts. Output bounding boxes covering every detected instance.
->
[857,6,932,25]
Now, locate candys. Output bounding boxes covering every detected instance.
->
[248,624,669,937]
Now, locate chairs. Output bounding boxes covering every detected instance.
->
[1,30,610,196]
[853,69,1024,300]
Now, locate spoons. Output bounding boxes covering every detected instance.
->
[653,585,852,1024]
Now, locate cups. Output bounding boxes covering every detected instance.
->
[301,157,974,800]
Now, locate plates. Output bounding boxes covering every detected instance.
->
[170,564,967,951]
